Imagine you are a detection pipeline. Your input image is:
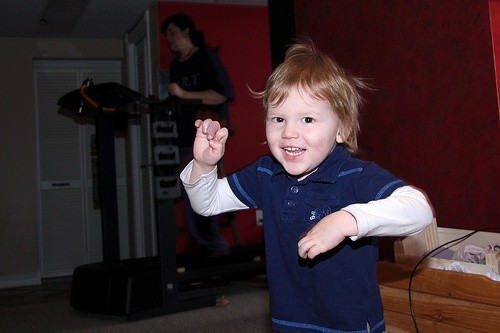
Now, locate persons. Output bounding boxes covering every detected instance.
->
[180,43,434,333]
[157,14,235,288]
